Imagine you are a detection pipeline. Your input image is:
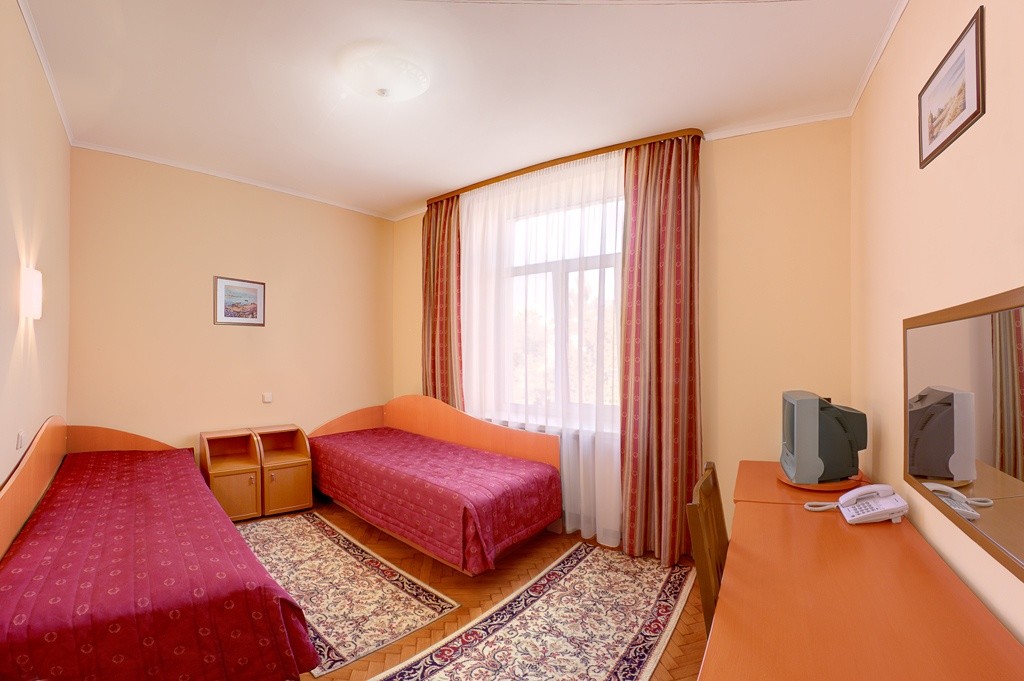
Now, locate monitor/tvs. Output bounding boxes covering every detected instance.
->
[779,390,868,484]
[908,384,978,481]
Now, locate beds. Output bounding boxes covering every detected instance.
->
[0,415,320,681]
[307,394,560,577]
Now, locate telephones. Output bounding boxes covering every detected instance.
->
[922,482,980,521]
[838,484,908,525]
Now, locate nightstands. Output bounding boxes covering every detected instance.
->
[200,422,312,521]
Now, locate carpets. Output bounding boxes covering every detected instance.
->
[366,542,696,681]
[236,513,463,678]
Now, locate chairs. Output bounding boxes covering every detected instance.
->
[685,462,728,636]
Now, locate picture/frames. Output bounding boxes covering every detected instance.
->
[213,276,265,326]
[917,5,985,169]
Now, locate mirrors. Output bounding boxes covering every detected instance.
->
[902,285,1024,582]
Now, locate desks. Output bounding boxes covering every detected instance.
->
[698,460,1024,681]
[918,458,1024,563]
